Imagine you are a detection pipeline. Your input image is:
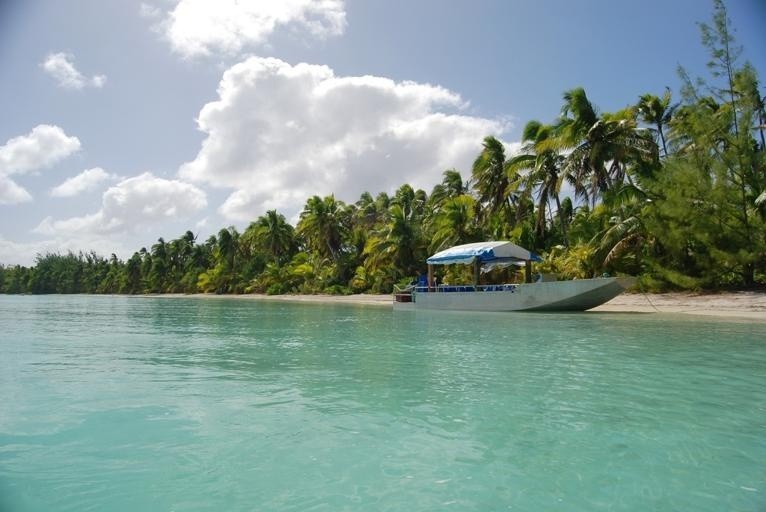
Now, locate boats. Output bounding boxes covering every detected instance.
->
[392,240,632,314]
